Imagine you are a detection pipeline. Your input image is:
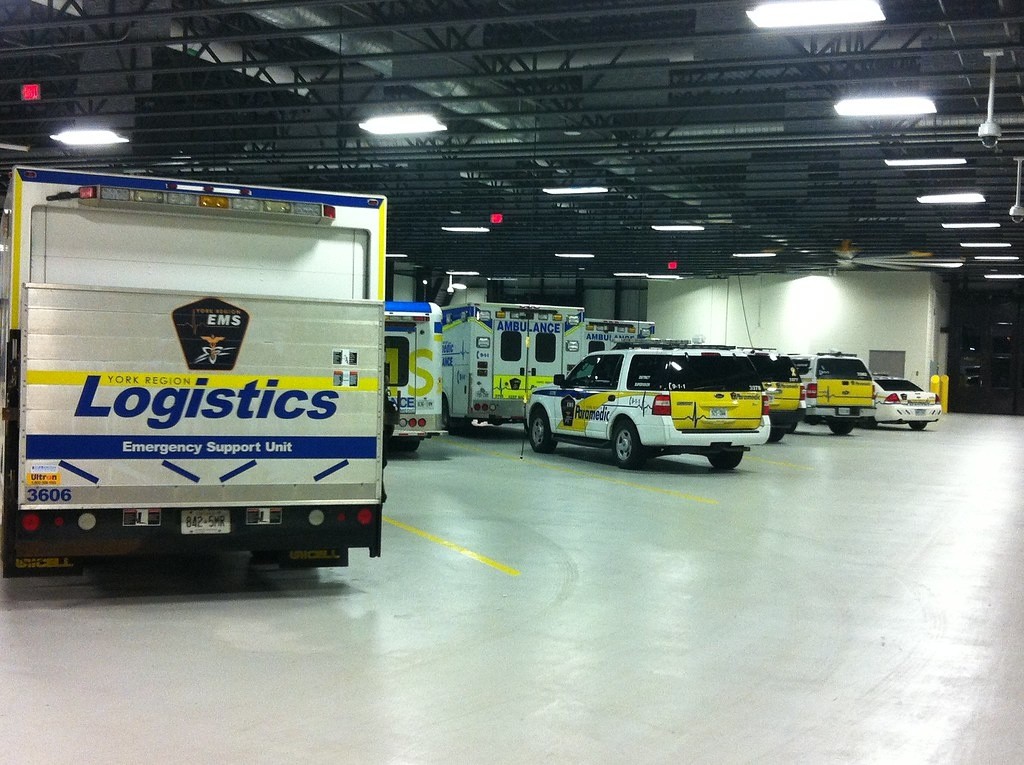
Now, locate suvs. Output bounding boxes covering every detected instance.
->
[524,339,772,470]
[786,350,874,437]
[737,345,807,444]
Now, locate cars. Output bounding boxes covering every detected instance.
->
[872,372,942,430]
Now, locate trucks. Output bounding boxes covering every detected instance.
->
[440,301,585,427]
[2,162,387,576]
[384,300,443,451]
[584,316,657,359]
